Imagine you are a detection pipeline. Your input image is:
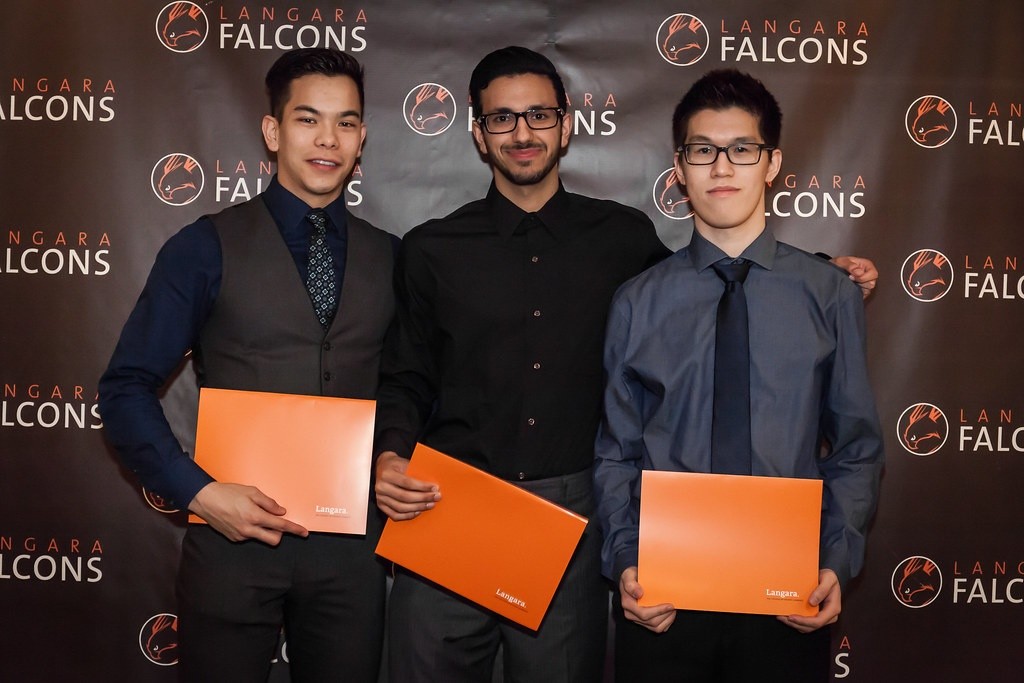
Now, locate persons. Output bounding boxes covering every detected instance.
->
[97,46,401,683]
[592,68,887,683]
[373,45,877,683]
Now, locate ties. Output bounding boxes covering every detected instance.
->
[709,257,755,476]
[306,207,338,334]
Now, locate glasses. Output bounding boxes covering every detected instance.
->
[477,107,566,134]
[676,143,775,165]
